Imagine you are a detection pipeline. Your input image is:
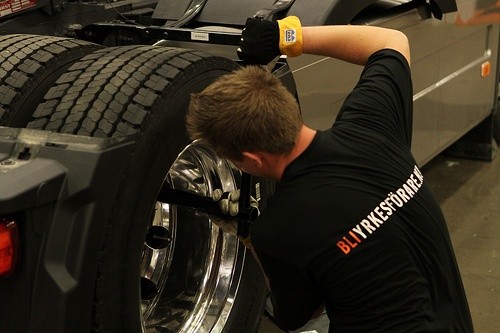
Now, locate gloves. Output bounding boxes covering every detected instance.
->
[209,189,259,249]
[237,16,304,65]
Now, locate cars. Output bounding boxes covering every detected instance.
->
[140,0,500,171]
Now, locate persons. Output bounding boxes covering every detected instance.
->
[188,16,473,333]
